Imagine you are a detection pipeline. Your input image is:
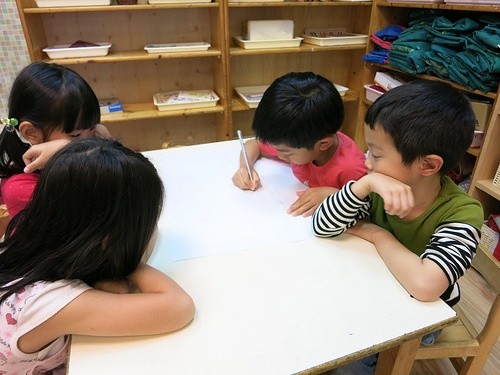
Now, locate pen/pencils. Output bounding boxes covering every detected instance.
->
[236,129,256,192]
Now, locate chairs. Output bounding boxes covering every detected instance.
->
[374,246,500,375]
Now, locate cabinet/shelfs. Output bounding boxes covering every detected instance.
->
[15,0,500,292]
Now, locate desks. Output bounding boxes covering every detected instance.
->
[66,141,458,375]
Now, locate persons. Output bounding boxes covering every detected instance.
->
[232,73,367,217]
[0,138,195,375]
[0,62,112,247]
[313,80,483,375]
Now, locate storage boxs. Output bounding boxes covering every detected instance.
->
[301,34,368,47]
[42,42,112,59]
[364,84,384,102]
[232,36,305,49]
[152,90,220,112]
[234,83,349,108]
[471,100,492,132]
